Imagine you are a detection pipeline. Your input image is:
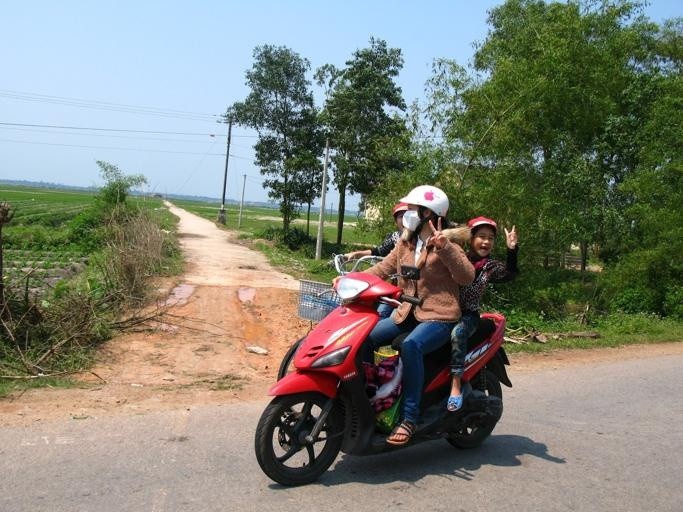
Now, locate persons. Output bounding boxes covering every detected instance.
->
[331,185,476,446]
[339,202,411,321]
[445,214,519,414]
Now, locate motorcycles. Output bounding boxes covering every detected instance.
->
[254,265,512,488]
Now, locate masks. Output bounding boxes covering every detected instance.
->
[401,210,422,233]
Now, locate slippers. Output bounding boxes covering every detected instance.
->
[447,388,465,412]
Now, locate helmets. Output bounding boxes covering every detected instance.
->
[398,184,450,218]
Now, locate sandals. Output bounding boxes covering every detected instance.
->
[386,419,417,446]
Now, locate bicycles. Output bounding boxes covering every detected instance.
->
[278,254,386,383]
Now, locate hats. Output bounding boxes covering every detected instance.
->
[392,202,409,217]
[466,216,497,232]
